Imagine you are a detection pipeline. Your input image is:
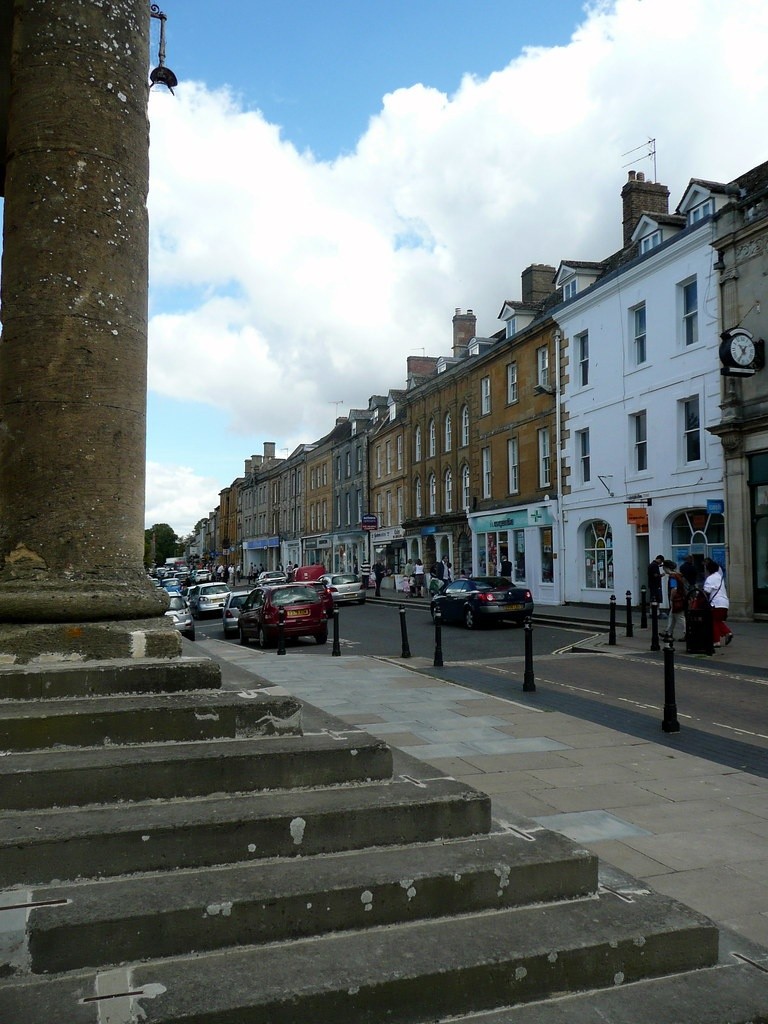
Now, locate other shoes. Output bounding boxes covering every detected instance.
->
[725,633,733,646]
[678,635,686,641]
[659,631,668,636]
[713,641,720,648]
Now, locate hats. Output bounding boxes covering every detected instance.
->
[663,560,676,569]
[229,563,234,566]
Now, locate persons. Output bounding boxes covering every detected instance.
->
[374,559,384,596]
[312,560,326,574]
[250,562,263,581]
[217,564,241,584]
[659,555,697,640]
[702,558,733,647]
[460,569,466,578]
[360,559,371,589]
[430,555,452,594]
[277,562,283,571]
[403,559,425,599]
[498,556,513,577]
[287,561,292,571]
[647,555,667,619]
[293,564,299,582]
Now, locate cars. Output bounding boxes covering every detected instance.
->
[145,561,213,600]
[429,576,534,631]
[218,590,252,638]
[316,572,367,606]
[254,571,288,587]
[162,588,196,642]
[237,584,328,649]
[286,581,335,617]
[188,582,234,620]
[289,565,325,581]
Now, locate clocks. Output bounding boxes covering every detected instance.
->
[731,335,756,365]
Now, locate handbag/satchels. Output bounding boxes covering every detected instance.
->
[429,578,444,594]
[673,593,684,614]
[409,577,415,587]
[371,571,377,581]
[403,577,410,592]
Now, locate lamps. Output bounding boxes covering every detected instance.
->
[534,384,555,394]
[598,474,615,497]
[473,497,477,509]
